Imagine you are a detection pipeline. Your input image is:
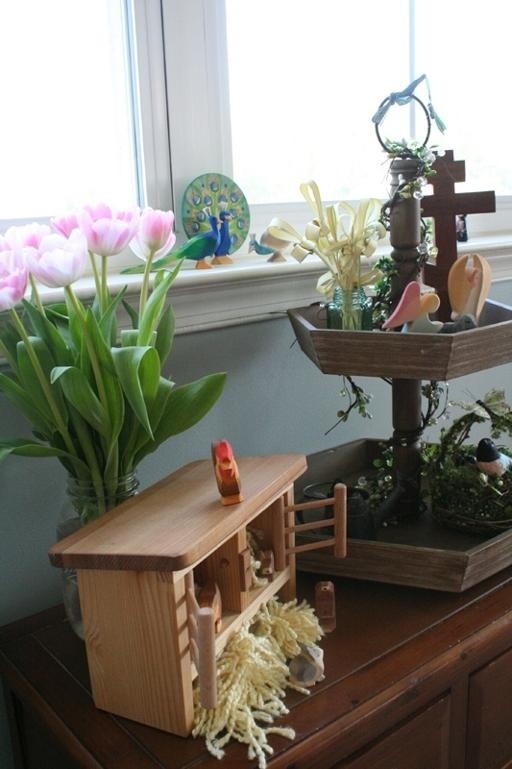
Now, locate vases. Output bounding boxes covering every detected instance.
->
[321,287,367,332]
[52,474,139,641]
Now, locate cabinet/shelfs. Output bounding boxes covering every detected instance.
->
[47,452,347,738]
[4,569,512,768]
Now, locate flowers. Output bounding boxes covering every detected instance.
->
[0,203,227,526]
[260,180,386,332]
